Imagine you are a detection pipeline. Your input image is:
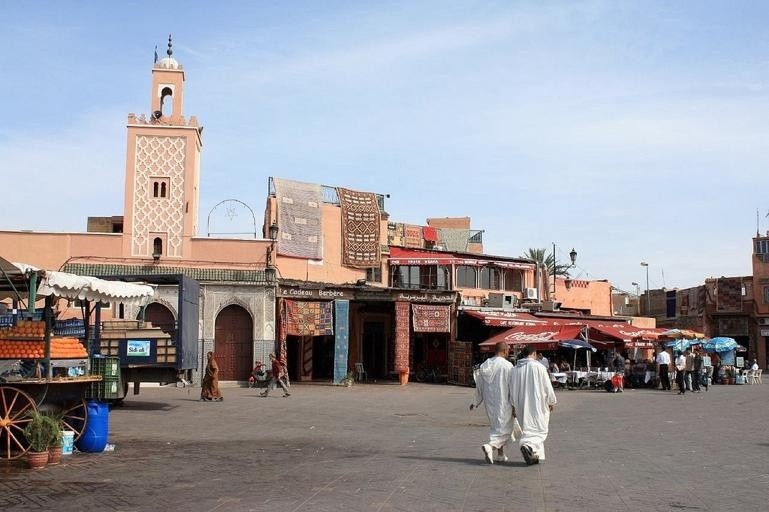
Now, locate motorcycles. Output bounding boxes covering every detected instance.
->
[247,360,278,388]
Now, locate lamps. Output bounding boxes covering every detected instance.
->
[152,251,160,263]
[564,276,573,291]
[269,221,278,239]
[570,248,576,264]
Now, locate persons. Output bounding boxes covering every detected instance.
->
[508,346,558,465]
[200,352,224,402]
[613,345,759,395]
[40,300,61,330]
[259,354,291,397]
[470,341,516,464]
[538,353,571,373]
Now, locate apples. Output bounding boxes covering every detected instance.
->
[0,320,88,359]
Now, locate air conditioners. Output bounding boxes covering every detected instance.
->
[757,317,769,327]
[523,287,538,300]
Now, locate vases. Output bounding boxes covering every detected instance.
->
[722,379,729,384]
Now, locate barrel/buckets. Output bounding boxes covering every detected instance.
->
[74,400,109,452]
[59,430,76,455]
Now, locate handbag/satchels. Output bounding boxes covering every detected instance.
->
[612,374,623,386]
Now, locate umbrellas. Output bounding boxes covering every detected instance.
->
[557,339,597,371]
[703,336,738,353]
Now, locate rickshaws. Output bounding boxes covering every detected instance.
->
[0,372,103,461]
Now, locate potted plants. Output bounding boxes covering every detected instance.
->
[47,414,65,465]
[23,409,50,469]
[727,369,735,384]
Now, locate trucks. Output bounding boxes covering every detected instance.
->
[59,274,203,411]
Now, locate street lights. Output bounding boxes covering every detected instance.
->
[632,281,640,316]
[641,261,651,318]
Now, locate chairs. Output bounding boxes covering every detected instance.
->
[735,368,763,385]
[355,363,368,384]
[548,366,616,390]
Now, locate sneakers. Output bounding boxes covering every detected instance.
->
[659,385,701,396]
[260,392,268,397]
[519,445,540,465]
[283,393,290,398]
[482,443,495,464]
[496,453,508,462]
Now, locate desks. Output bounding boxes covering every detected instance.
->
[635,370,673,390]
